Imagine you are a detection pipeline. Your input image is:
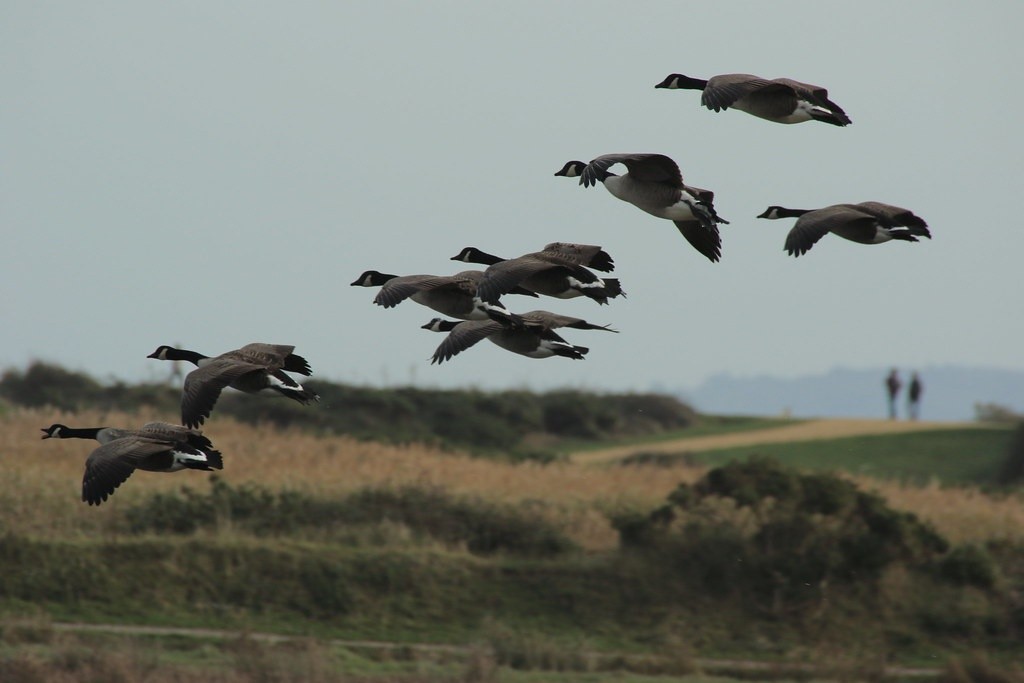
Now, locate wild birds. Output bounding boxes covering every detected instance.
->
[349,242,627,366]
[147,342,321,430]
[40,422,223,507]
[654,73,853,127]
[757,202,931,259]
[554,153,730,264]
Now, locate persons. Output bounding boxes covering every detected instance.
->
[904,367,924,425]
[883,365,900,421]
